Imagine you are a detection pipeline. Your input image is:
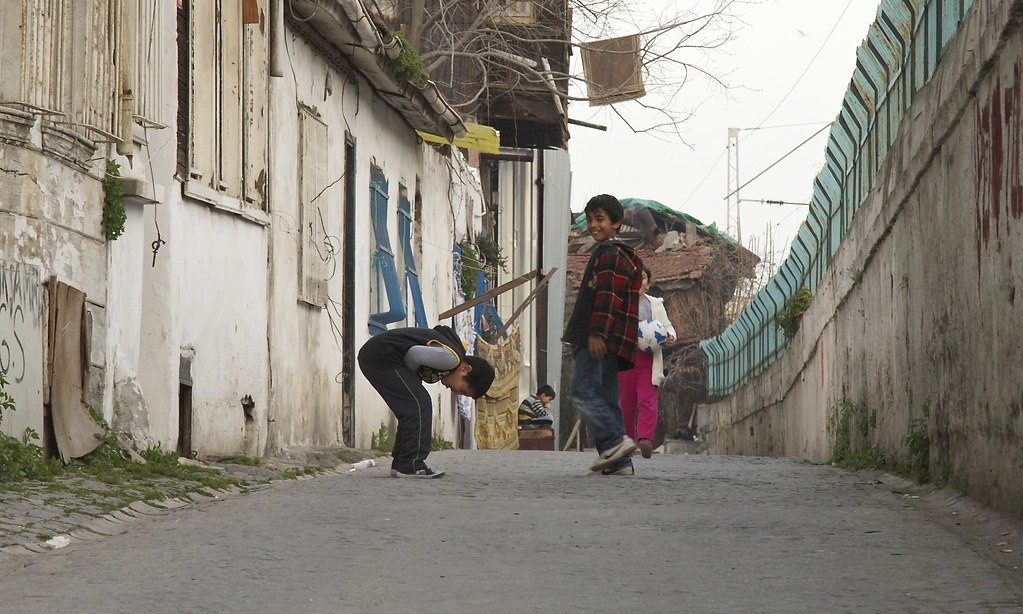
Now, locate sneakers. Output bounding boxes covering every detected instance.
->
[391,458,445,479]
[591,436,636,472]
[588,465,635,476]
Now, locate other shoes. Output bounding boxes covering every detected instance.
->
[640,440,653,459]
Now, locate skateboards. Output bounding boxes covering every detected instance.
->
[600,454,635,476]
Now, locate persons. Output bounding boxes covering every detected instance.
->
[615,264,677,458]
[358,325,495,478]
[563,194,643,477]
[518,384,556,429]
